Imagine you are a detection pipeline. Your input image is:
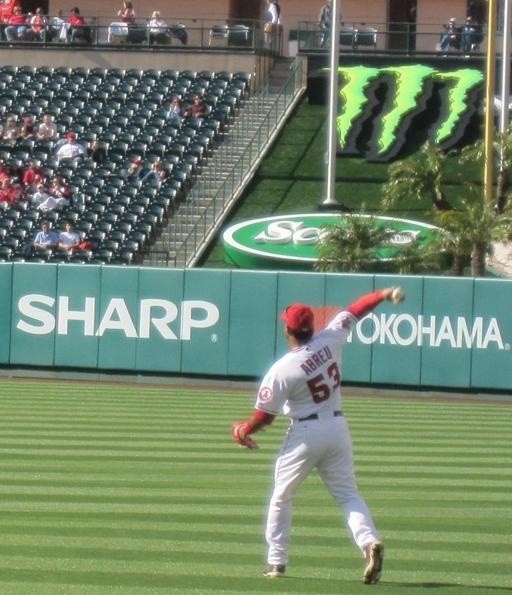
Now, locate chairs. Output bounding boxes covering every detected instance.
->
[208,24,249,47]
[1,65,252,266]
[338,26,377,49]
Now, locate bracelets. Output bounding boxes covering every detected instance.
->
[243,422,255,435]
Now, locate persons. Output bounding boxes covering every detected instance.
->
[0,0,95,49]
[319,0,333,48]
[165,97,188,122]
[263,0,282,50]
[114,1,136,25]
[0,112,106,258]
[435,16,461,52]
[182,93,210,128]
[146,10,169,45]
[459,16,484,52]
[234,283,405,585]
[127,156,168,186]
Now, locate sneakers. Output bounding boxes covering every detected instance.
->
[362,541,385,585]
[263,564,286,577]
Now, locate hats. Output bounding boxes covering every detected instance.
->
[280,304,316,336]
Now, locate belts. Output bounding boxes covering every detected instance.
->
[299,410,344,421]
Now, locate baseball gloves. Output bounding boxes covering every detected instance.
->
[231,420,267,449]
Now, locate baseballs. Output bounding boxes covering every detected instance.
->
[390,287,402,300]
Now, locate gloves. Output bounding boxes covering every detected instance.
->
[232,421,259,450]
